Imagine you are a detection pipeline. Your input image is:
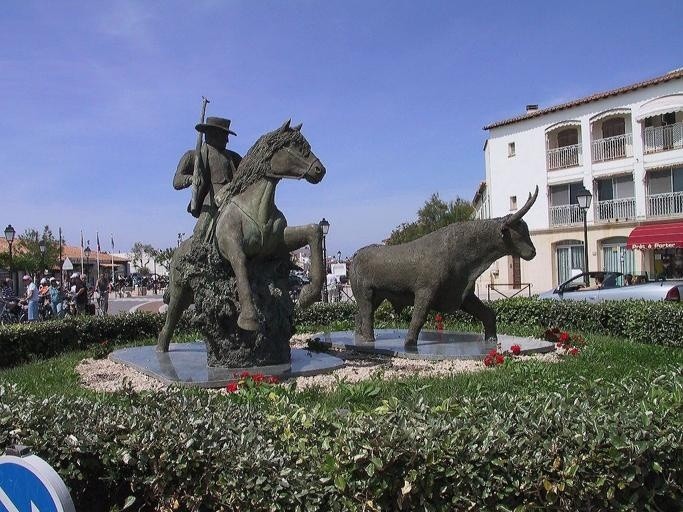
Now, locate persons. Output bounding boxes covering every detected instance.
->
[624,274,632,286]
[327,268,337,303]
[0,274,111,323]
[173,117,242,240]
[578,273,604,290]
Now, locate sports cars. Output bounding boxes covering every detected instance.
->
[533,272,683,306]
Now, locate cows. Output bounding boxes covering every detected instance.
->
[346,184,540,348]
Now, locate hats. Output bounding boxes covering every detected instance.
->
[69,272,80,278]
[23,275,32,282]
[49,277,54,283]
[39,278,48,285]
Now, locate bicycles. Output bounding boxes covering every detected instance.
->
[0,292,94,326]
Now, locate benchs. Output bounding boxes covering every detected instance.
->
[116,284,147,298]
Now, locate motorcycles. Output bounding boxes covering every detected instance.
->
[105,270,169,290]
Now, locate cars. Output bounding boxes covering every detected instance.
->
[287,271,310,298]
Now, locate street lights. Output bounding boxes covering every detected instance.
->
[82,246,94,291]
[574,184,594,292]
[4,223,18,297]
[35,236,46,292]
[316,218,333,307]
[336,250,344,265]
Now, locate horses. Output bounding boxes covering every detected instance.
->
[155,117,326,355]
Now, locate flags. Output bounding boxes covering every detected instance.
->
[97,237,100,252]
[81,235,83,247]
[112,238,114,247]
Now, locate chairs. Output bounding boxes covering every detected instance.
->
[599,273,617,289]
